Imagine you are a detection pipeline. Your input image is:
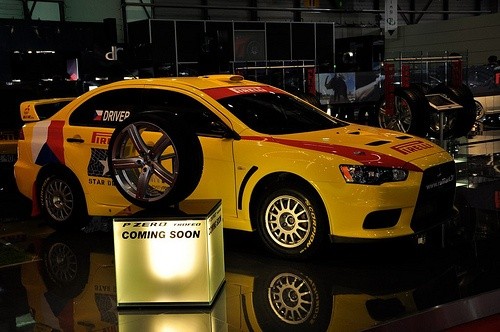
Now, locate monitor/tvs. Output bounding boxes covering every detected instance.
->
[424,93,456,108]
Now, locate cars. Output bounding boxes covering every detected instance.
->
[11,70,459,258]
